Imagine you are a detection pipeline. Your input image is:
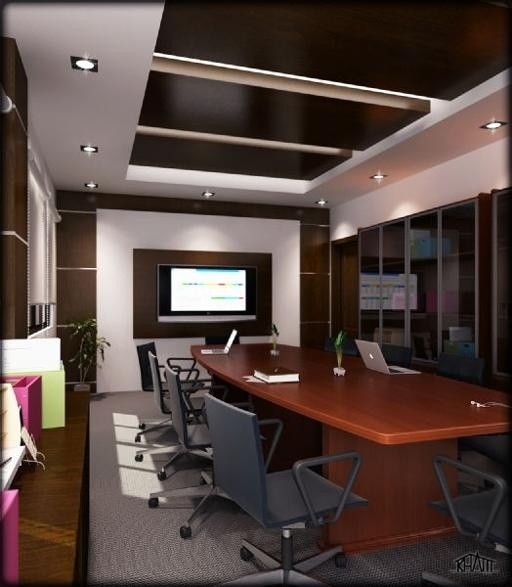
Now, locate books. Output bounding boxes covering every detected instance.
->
[252,366,300,384]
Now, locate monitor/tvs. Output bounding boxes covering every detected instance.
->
[360,273,418,311]
[157,264,257,323]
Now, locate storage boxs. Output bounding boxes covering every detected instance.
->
[1,375,42,444]
[2,488,19,585]
[2,360,65,429]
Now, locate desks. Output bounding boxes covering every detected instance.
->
[190,343,512,557]
[8,391,90,587]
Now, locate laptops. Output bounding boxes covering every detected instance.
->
[201,328,239,356]
[354,339,420,377]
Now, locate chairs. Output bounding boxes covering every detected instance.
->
[326,337,486,387]
[134,342,265,538]
[422,456,512,587]
[201,393,368,586]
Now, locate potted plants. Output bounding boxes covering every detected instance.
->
[333,330,347,377]
[69,318,111,391]
[269,324,280,356]
